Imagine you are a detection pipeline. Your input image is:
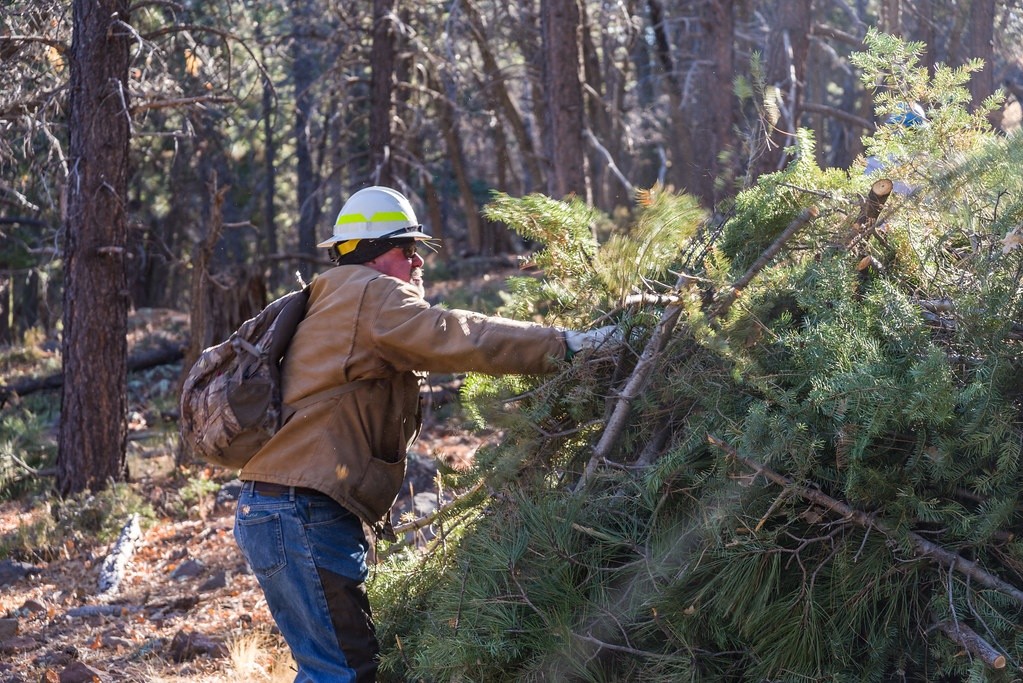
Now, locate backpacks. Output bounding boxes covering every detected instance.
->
[181,278,385,470]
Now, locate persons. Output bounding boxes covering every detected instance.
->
[233,184,648,683]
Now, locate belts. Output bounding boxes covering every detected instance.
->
[243,480,330,498]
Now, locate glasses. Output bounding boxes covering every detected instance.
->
[395,240,417,259]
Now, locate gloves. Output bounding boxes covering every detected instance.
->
[563,323,646,362]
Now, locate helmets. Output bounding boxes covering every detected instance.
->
[316,185,432,248]
